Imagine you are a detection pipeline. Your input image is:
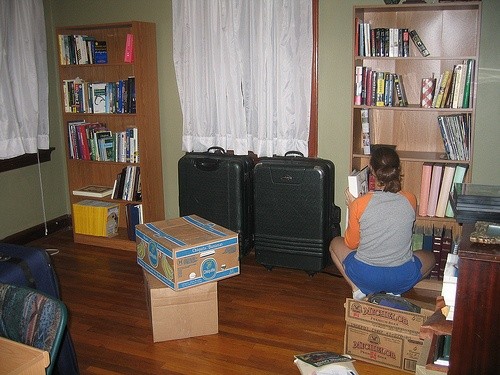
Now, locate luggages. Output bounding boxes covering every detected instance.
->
[0,244,78,375]
[177,147,252,260]
[253,149,339,275]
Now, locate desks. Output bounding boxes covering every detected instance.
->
[0,336,50,375]
[447,224,500,375]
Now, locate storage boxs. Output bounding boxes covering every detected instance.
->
[135,215,240,292]
[142,269,219,343]
[344,298,436,375]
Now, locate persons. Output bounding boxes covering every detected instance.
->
[329,147,438,299]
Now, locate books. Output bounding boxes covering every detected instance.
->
[57,34,132,65]
[355,17,429,58]
[67,121,140,164]
[63,75,136,115]
[73,165,143,241]
[347,61,473,285]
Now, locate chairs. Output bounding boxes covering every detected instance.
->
[0,282,67,375]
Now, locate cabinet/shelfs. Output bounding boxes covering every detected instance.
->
[352,2,482,291]
[55,21,165,252]
